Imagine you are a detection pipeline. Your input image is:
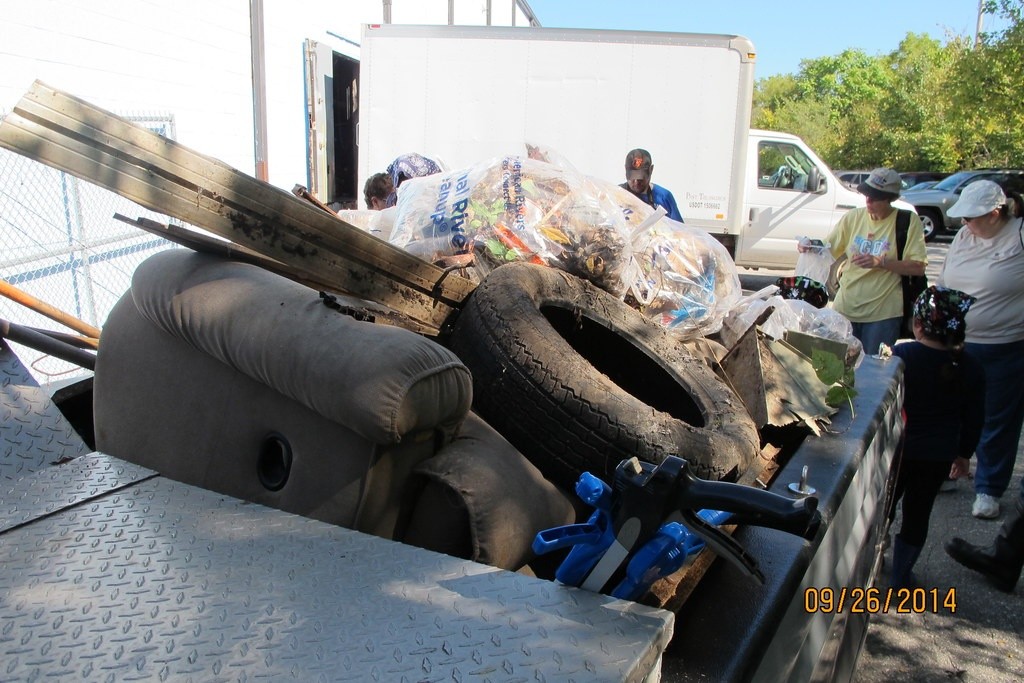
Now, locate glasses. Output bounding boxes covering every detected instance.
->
[964,213,991,221]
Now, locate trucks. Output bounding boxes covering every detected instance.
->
[358,23,920,271]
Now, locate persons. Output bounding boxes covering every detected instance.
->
[943,481,1024,593]
[364,153,441,210]
[936,181,1024,518]
[798,168,928,355]
[619,149,685,222]
[880,286,983,582]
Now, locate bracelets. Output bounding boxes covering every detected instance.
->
[881,258,884,266]
[811,239,822,254]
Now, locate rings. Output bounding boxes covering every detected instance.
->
[863,263,864,266]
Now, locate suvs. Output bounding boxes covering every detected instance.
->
[833,168,1024,244]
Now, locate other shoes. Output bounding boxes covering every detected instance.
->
[945,537,1021,594]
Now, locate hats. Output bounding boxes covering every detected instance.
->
[856,168,902,201]
[626,149,652,181]
[946,180,1007,217]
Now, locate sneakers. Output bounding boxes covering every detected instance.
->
[972,492,1000,519]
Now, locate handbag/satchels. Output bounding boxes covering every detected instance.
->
[895,209,928,320]
